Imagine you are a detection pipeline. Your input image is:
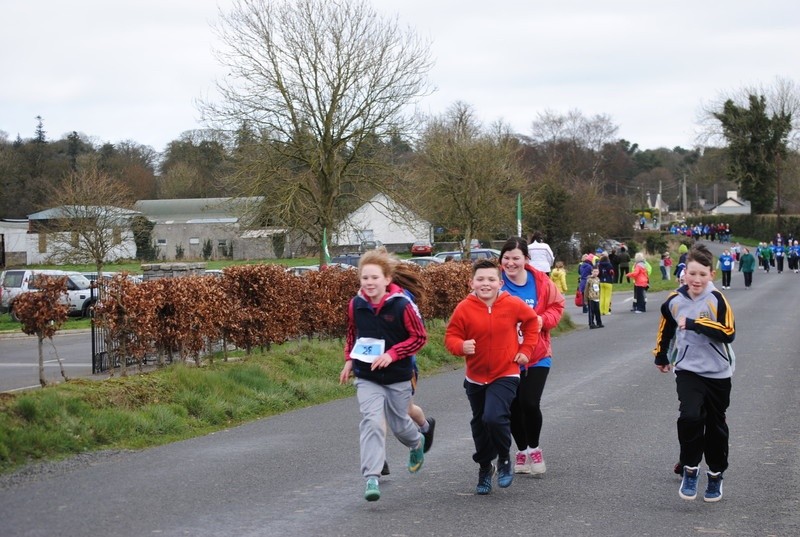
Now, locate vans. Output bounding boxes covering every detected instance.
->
[0,268,102,319]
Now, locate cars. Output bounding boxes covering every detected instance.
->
[82,270,143,285]
[412,240,434,257]
[358,240,388,256]
[286,248,503,275]
[452,237,493,252]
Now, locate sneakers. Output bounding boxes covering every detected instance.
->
[496,457,513,488]
[363,479,380,501]
[408,432,424,473]
[704,467,723,502]
[477,463,496,495]
[528,447,546,475]
[513,448,530,473]
[679,466,701,501]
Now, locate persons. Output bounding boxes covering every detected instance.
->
[526,231,689,329]
[730,242,741,263]
[498,237,565,475]
[445,260,539,493]
[738,248,755,290]
[445,256,454,262]
[639,213,733,245]
[716,248,735,289]
[652,244,736,503]
[340,247,436,501]
[755,233,800,273]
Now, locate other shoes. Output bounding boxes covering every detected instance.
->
[745,286,749,290]
[590,325,598,329]
[630,308,637,311]
[635,310,643,313]
[722,286,726,290]
[759,266,763,269]
[795,269,799,273]
[727,286,731,290]
[597,324,604,328]
[777,270,781,273]
[381,461,389,475]
[605,312,611,315]
[417,418,435,452]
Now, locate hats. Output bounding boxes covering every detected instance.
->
[596,247,602,253]
[678,244,687,254]
